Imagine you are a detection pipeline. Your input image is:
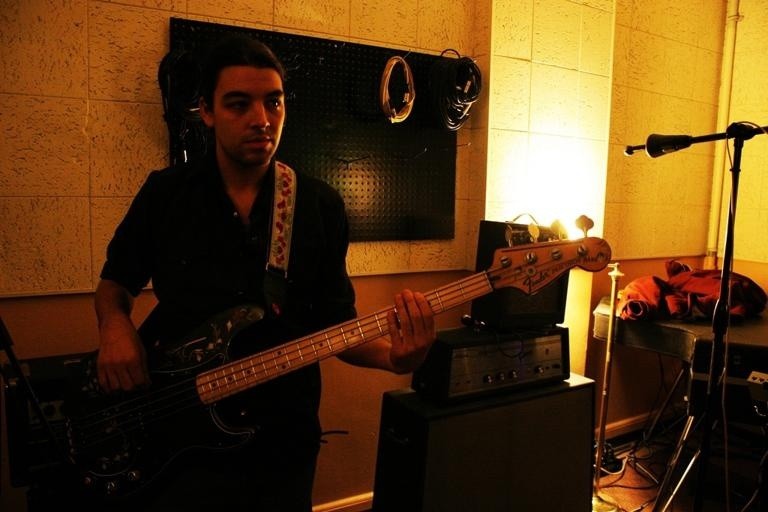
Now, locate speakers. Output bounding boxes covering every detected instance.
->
[371,372,596,511]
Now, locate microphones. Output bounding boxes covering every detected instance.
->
[624,133,692,160]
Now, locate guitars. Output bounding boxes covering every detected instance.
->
[67,214,610,498]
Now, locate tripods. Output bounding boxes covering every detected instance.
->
[650,122,768,512]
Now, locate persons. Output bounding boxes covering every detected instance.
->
[94,42,436,512]
